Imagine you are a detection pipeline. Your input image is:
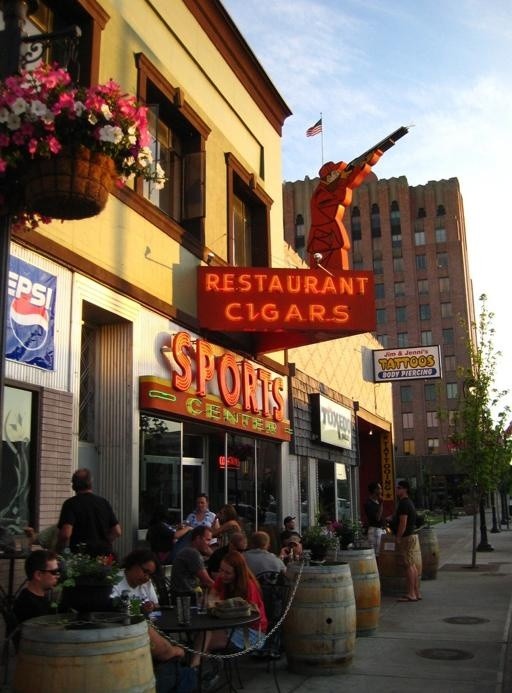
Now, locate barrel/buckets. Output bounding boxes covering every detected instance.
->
[338,548,382,632]
[418,527,440,581]
[381,533,423,588]
[15,610,156,692]
[286,561,356,673]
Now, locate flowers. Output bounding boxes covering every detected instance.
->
[0,60,168,189]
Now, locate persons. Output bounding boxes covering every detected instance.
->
[108,492,302,692]
[58,468,122,555]
[367,482,385,558]
[395,480,422,602]
[8,550,61,652]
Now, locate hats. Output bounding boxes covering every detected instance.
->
[288,535,301,545]
[284,516,296,524]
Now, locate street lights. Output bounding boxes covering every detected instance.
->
[459,370,495,553]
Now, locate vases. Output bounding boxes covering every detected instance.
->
[26,144,115,220]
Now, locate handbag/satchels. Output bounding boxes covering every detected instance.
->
[208,596,260,619]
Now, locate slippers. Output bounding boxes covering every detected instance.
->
[396,595,421,602]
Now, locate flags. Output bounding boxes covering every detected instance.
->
[305,118,322,137]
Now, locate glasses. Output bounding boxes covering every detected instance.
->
[139,564,155,578]
[38,568,61,575]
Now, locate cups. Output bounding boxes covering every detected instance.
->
[195,587,209,615]
[176,597,191,624]
